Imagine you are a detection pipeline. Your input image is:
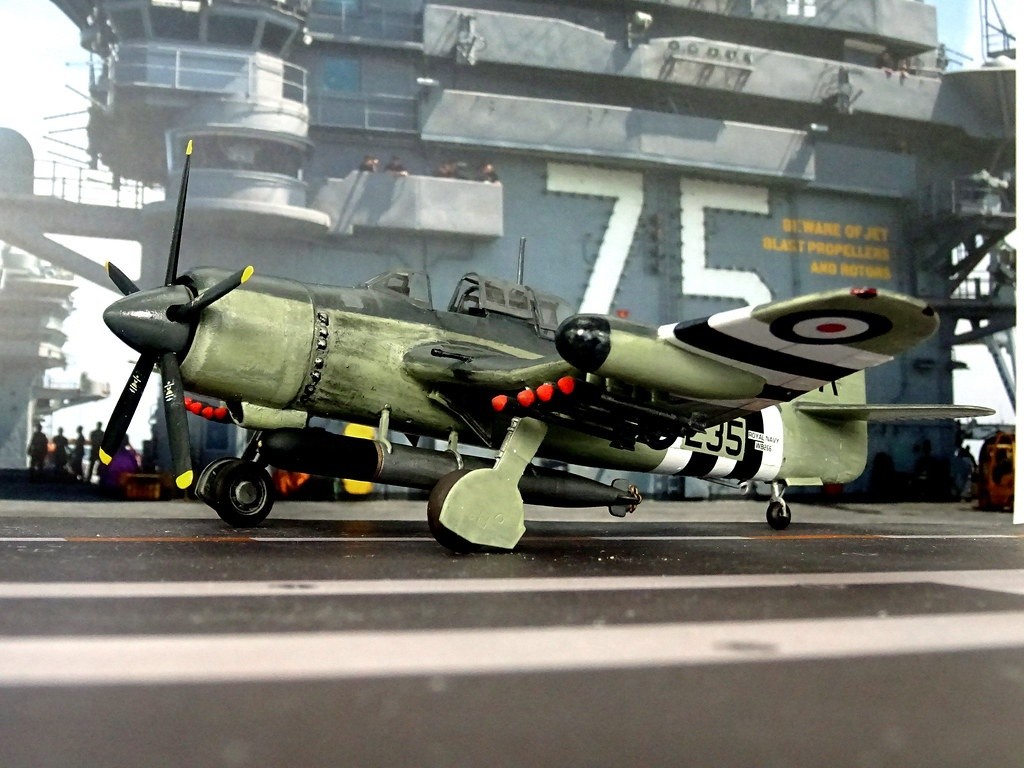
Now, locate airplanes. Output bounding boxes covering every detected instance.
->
[98,142,995,555]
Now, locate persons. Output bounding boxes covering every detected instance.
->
[88,421,105,481]
[360,155,375,171]
[441,161,475,179]
[385,154,407,175]
[53,426,71,468]
[481,164,501,185]
[27,424,47,469]
[881,53,909,77]
[73,426,85,479]
